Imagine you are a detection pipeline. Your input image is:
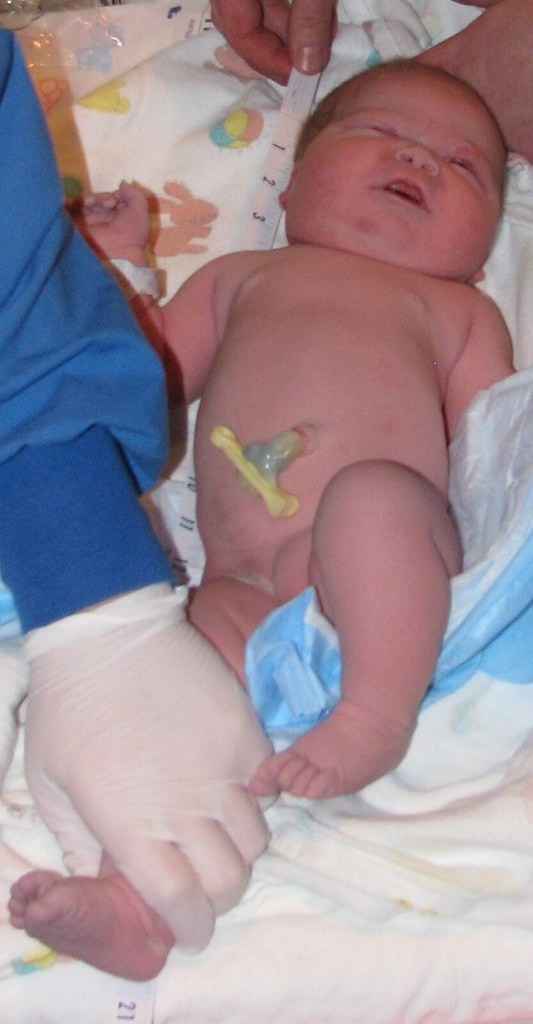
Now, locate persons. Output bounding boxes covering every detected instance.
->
[1,1,533,982]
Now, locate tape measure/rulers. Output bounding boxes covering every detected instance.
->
[105,64,326,1024]
